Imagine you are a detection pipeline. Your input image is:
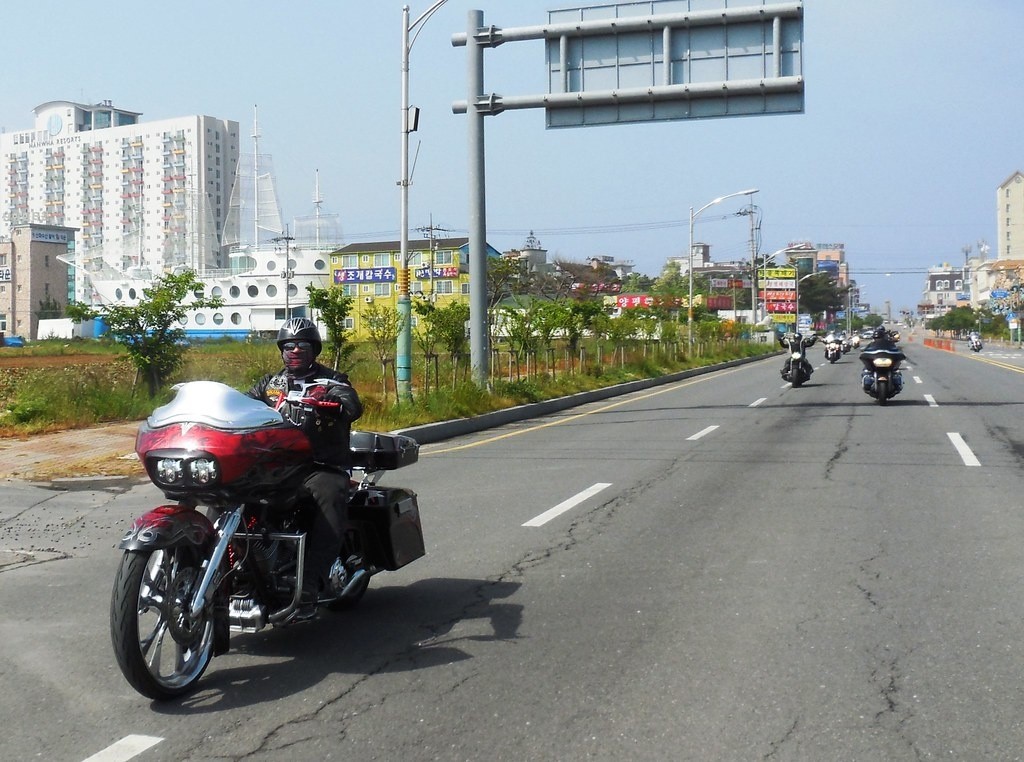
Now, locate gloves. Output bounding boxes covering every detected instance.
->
[315,393,343,418]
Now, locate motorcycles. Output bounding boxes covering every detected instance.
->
[856,337,907,406]
[818,332,844,364]
[779,336,815,388]
[967,331,983,352]
[105,380,422,706]
[838,334,861,355]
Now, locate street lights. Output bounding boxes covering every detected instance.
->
[754,243,807,324]
[846,283,867,334]
[689,188,760,355]
[783,260,828,332]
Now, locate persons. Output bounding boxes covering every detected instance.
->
[841,331,852,352]
[778,333,817,375]
[861,325,901,370]
[243,317,364,618]
[966,328,984,349]
[823,331,842,359]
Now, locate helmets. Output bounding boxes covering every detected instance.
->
[793,333,802,341]
[841,332,845,336]
[876,325,885,331]
[831,330,836,333]
[276,317,322,357]
[872,330,884,338]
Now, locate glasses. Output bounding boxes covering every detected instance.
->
[282,342,311,351]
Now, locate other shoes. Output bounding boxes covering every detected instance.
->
[314,574,349,602]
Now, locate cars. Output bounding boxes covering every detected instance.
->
[862,331,873,340]
